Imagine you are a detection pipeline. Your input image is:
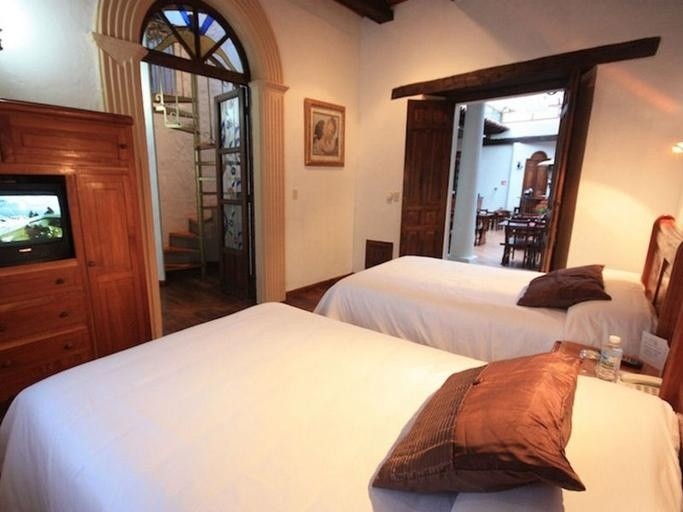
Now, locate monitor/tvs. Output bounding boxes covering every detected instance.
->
[0,182,73,268]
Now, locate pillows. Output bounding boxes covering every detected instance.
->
[370,352,587,497]
[517,263,610,309]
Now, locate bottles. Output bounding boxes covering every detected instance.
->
[598,335,623,381]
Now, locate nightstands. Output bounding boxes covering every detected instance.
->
[550,337,662,382]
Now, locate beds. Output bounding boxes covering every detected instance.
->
[13,298,683,512]
[326,214,681,358]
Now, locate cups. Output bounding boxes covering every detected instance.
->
[576,347,600,377]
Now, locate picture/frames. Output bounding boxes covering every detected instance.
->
[303,97,346,168]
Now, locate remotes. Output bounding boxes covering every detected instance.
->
[598,349,644,368]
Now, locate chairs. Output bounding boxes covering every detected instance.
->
[498,206,548,269]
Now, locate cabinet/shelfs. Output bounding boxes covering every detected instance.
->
[0,98,156,410]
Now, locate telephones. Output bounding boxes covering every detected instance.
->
[616,370,662,397]
